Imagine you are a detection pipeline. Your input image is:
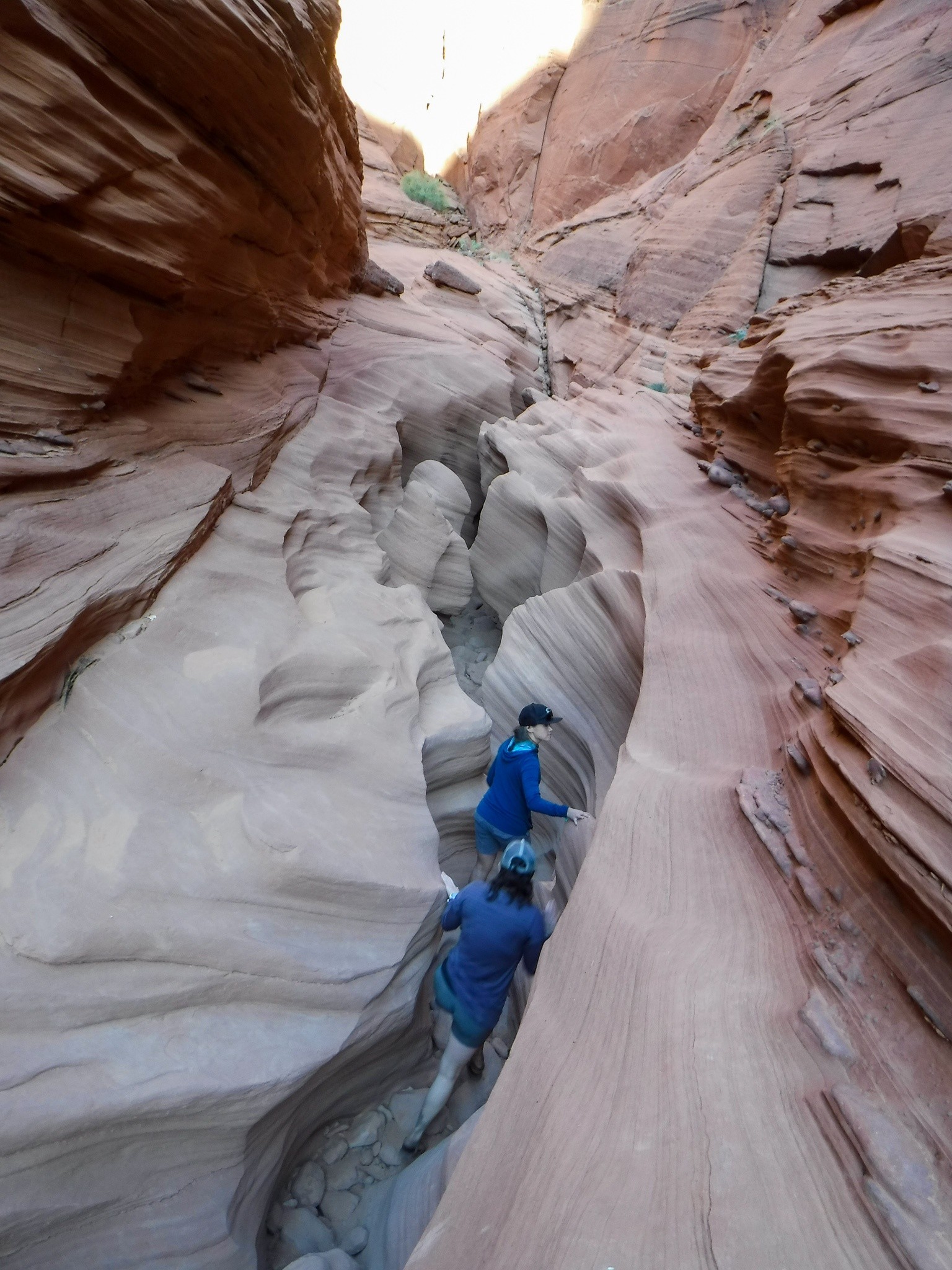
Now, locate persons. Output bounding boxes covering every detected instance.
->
[401,837,549,1156]
[468,702,594,884]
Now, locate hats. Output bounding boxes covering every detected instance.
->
[518,702,563,727]
[500,838,536,874]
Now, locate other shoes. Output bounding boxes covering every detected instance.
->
[471,1054,486,1076]
[400,1136,427,1157]
